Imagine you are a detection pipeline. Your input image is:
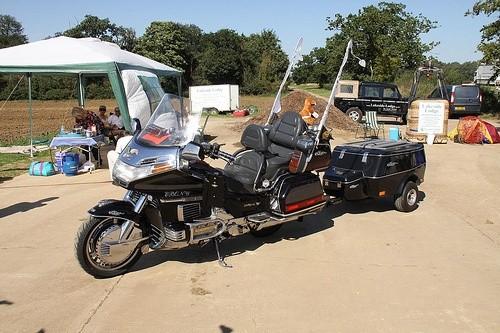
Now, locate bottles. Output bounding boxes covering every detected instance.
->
[86,124,97,138]
[61,126,64,133]
[55,152,66,172]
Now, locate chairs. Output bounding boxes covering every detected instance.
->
[355,111,385,140]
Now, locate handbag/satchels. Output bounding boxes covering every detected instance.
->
[28,160,56,176]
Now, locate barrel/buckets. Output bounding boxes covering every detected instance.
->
[390,128,399,140]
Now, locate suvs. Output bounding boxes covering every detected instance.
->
[334,79,412,123]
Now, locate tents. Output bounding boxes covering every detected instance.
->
[0,36,184,158]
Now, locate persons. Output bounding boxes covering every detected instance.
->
[97,106,129,140]
[72,107,105,167]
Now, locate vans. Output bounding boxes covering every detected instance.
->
[426,83,482,117]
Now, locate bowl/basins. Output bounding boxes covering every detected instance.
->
[73,128,82,134]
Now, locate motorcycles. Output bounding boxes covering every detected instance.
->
[72,93,335,276]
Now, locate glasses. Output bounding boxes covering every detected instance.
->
[99,108,106,111]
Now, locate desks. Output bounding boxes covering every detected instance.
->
[50,137,101,174]
